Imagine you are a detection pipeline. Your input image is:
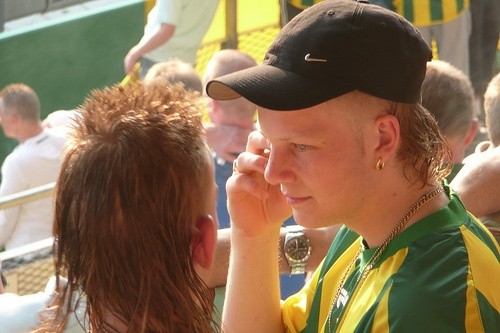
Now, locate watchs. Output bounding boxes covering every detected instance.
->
[283,223,313,276]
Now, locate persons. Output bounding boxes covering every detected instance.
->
[421,58,479,187]
[447,143,498,216]
[392,0,472,76]
[143,58,204,138]
[200,47,308,302]
[1,83,78,254]
[204,1,500,333]
[33,77,342,333]
[476,72,499,244]
[123,1,223,84]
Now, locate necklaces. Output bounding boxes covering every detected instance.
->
[328,183,443,333]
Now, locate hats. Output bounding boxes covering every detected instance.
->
[205,0,433,110]
